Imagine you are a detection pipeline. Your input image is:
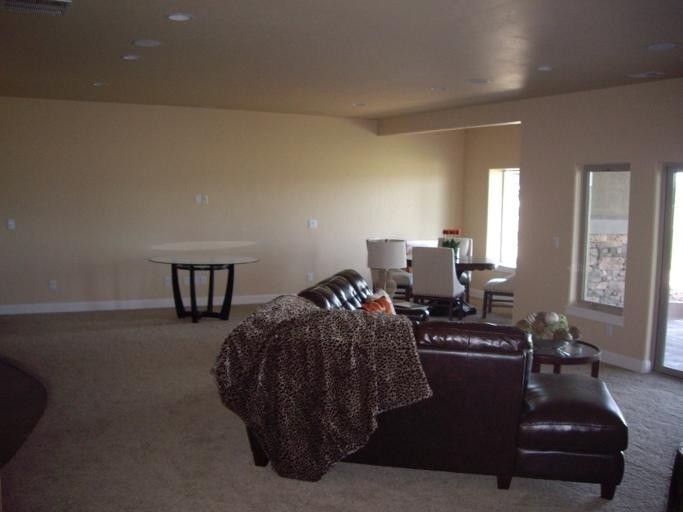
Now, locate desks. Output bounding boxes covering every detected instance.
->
[406,255,496,316]
[149,254,260,323]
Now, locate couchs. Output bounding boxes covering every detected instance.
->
[243,269,630,500]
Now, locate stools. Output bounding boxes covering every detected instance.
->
[482,272,515,320]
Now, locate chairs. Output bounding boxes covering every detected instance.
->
[366,237,473,321]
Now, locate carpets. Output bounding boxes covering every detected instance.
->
[1,303,683,510]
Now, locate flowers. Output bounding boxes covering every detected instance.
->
[441,228,462,258]
